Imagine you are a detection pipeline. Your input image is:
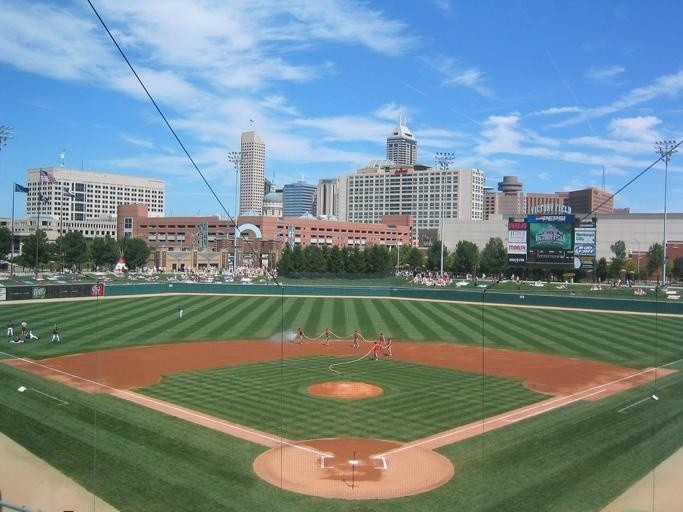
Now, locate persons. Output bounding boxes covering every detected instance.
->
[25,331,39,340]
[6,319,14,339]
[391,268,649,297]
[93,264,273,284]
[178,304,184,321]
[297,328,305,345]
[47,322,61,344]
[320,328,331,346]
[20,320,27,335]
[386,338,392,355]
[8,336,25,343]
[379,331,385,345]
[374,342,379,360]
[352,330,360,350]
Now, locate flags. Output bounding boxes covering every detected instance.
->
[13,182,29,193]
[63,187,78,199]
[40,170,57,184]
